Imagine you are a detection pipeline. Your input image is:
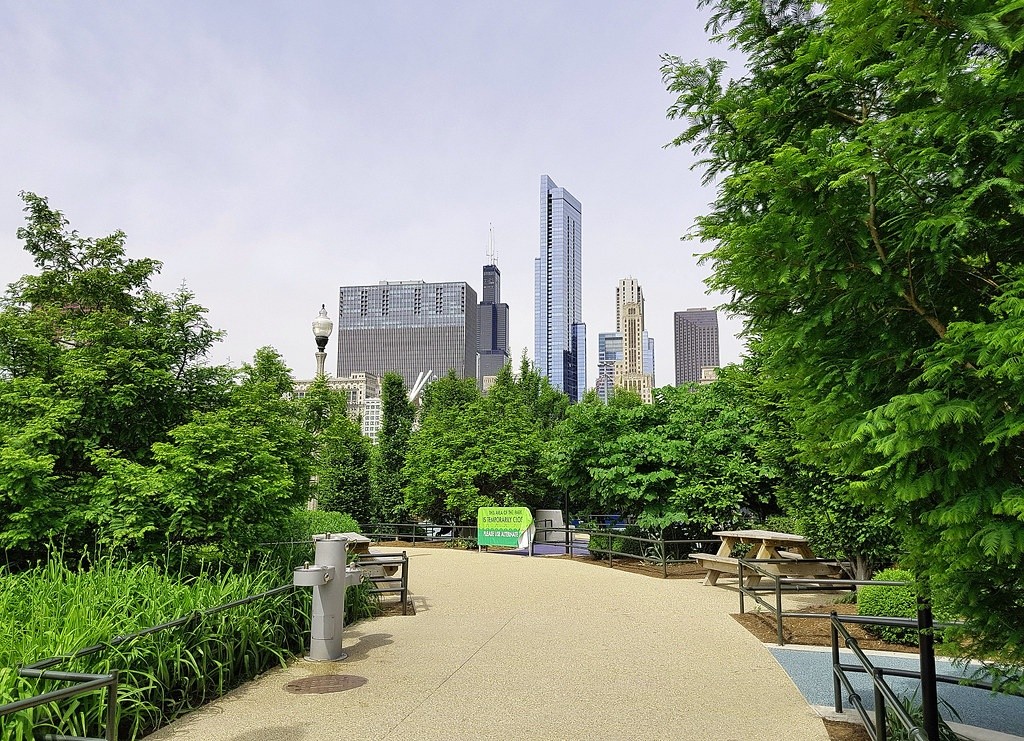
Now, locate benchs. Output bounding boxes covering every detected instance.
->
[689,551,851,576]
[359,553,401,579]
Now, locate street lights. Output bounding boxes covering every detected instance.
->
[308,303,334,510]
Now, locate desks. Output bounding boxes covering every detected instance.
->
[703,531,839,593]
[310,529,395,599]
